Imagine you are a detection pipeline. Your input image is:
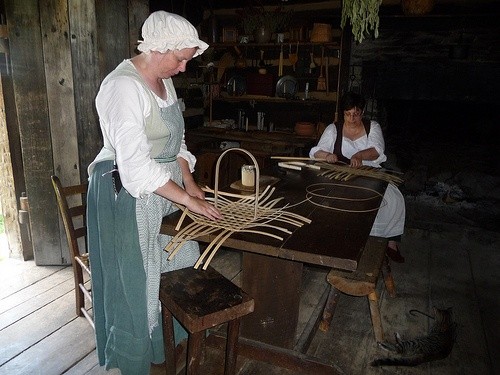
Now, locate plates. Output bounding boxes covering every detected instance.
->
[276,76,298,98]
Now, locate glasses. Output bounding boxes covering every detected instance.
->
[344,112,360,117]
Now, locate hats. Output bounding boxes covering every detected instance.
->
[137,10,210,58]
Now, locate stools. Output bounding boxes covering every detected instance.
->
[319,239,397,342]
[158,263,254,375]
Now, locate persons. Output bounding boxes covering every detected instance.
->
[85,11,223,375]
[309,93,406,264]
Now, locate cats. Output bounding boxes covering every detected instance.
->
[370,304,457,367]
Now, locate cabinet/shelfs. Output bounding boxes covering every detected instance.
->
[179,16,354,186]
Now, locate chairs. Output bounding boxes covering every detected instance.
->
[51,174,95,332]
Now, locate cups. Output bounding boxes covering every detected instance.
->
[242,37,248,44]
[242,165,255,186]
[295,122,315,136]
[278,34,284,42]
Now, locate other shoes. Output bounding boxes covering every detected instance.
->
[386,246,405,263]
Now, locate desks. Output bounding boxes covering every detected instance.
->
[161,168,394,375]
